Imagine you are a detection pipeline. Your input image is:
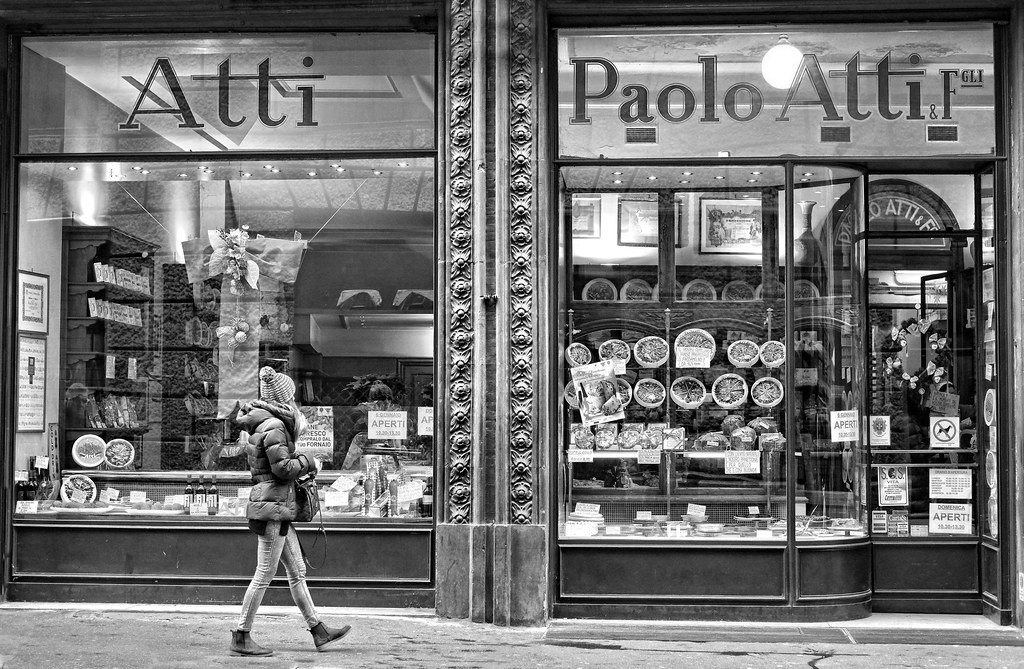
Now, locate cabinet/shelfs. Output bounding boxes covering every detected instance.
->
[59,225,162,442]
[565,367,784,452]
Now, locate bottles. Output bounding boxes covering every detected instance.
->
[185,474,194,514]
[348,476,365,512]
[196,474,206,503]
[209,474,219,515]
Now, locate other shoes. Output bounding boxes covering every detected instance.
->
[306,621,352,651]
[227,629,274,657]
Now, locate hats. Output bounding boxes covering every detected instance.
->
[367,380,395,403]
[259,366,296,406]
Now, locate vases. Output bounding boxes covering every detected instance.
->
[793,200,820,266]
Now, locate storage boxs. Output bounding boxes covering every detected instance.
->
[87,262,150,380]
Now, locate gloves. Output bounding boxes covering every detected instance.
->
[312,456,322,477]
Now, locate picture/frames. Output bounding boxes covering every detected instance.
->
[571,197,602,239]
[17,333,48,433]
[14,269,50,335]
[618,197,682,248]
[698,196,762,255]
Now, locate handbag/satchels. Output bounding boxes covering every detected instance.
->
[293,460,320,522]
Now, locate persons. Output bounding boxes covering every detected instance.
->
[346,379,402,469]
[64,383,89,428]
[229,366,351,658]
